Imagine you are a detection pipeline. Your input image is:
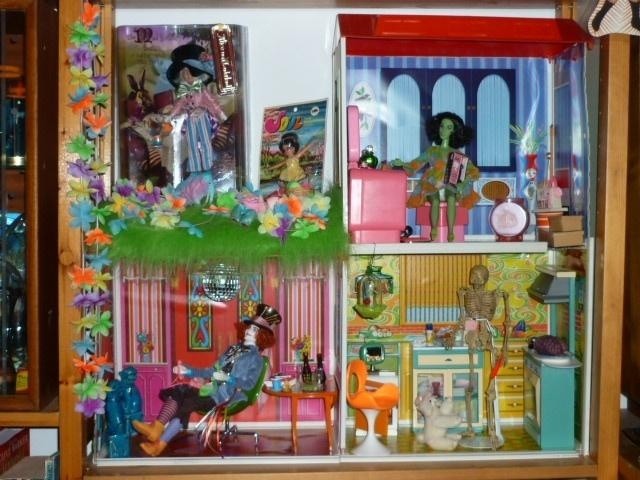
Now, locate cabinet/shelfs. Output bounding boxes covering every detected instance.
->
[0,0,59,412]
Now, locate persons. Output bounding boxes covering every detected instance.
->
[163,43,317,192]
[133,304,282,456]
[391,113,479,242]
[90,24,586,457]
